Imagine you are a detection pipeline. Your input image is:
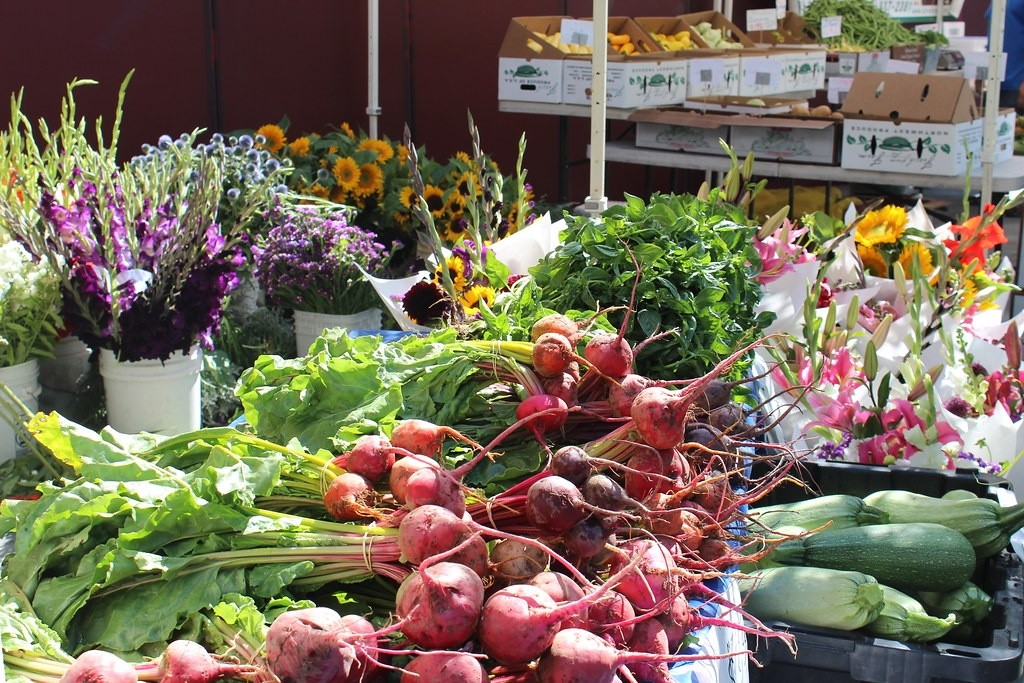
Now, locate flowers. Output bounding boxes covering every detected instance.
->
[0,69,536,372]
[691,133,1024,473]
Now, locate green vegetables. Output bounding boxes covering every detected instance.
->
[492,190,775,392]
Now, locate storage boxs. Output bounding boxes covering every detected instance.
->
[497,0,1016,177]
[741,460,1024,683]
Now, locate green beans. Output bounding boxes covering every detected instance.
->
[801,0,924,51]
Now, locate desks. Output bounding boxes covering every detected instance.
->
[588,140,1024,313]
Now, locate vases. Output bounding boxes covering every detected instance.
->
[37,335,96,429]
[0,359,43,462]
[98,346,203,434]
[293,309,383,357]
